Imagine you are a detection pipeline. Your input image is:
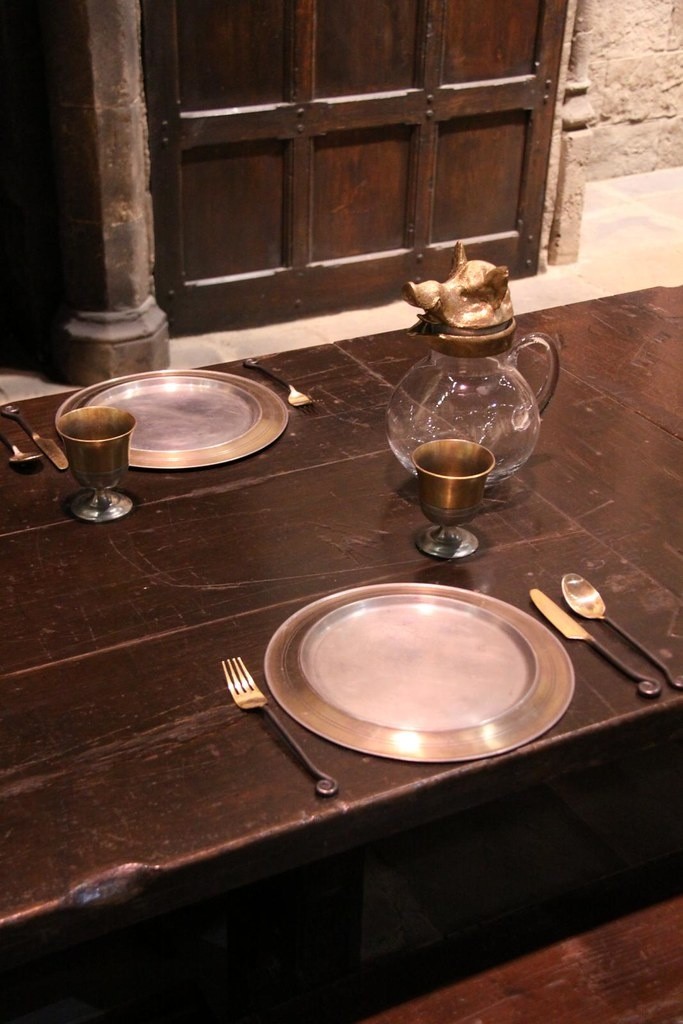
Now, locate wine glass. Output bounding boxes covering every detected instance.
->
[57,408,136,521]
[412,438,496,559]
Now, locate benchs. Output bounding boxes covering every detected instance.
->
[343,894,683,1024]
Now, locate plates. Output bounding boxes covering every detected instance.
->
[57,369,289,468]
[264,583,575,760]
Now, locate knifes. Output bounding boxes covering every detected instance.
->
[529,589,661,695]
[0,406,70,470]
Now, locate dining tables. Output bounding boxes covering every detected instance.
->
[0,285,683,932]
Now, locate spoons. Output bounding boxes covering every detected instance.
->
[0,431,43,462]
[561,572,683,688]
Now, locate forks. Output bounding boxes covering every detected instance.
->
[244,358,325,407]
[221,657,338,794]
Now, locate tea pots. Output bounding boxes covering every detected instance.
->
[388,241,559,485]
